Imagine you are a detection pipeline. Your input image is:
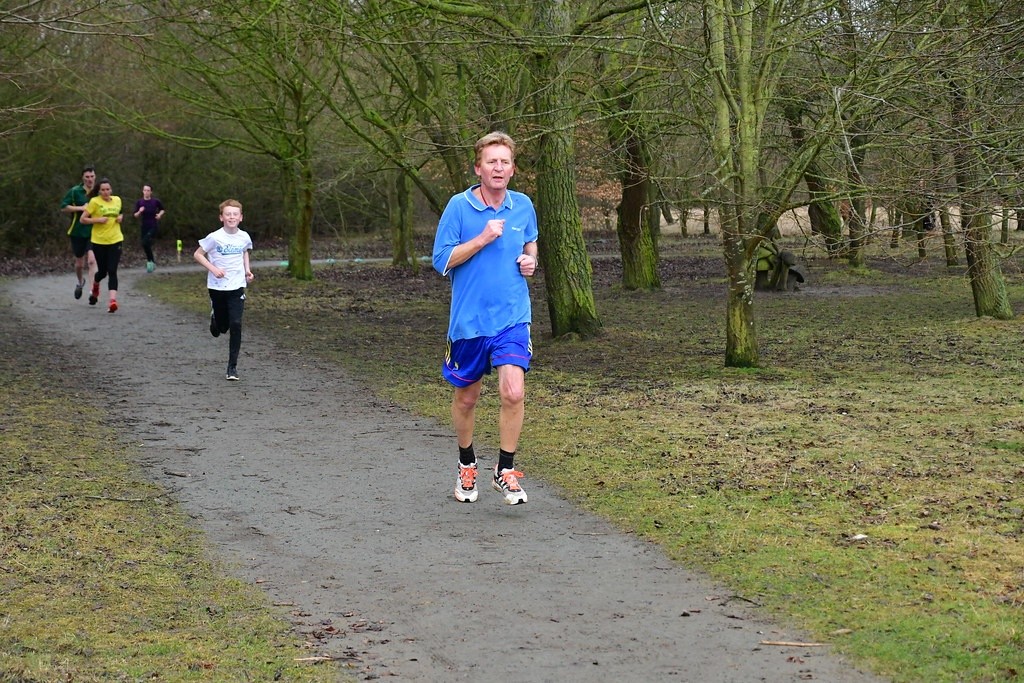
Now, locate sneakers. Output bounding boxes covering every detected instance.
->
[75,278,85,299]
[147,260,157,273]
[88,295,98,305]
[226,366,240,381]
[454,454,479,503]
[107,301,118,313]
[92,283,99,298]
[209,310,220,337]
[492,464,528,505]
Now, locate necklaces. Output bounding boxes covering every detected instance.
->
[480,185,490,207]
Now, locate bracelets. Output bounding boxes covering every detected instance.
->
[526,253,538,272]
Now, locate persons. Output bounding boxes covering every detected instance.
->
[79,178,124,313]
[133,184,165,274]
[431,130,538,507]
[59,167,96,302]
[194,199,255,381]
